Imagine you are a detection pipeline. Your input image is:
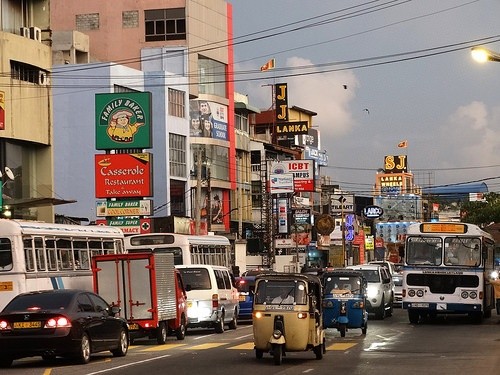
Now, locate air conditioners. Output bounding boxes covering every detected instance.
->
[36,70,46,85]
[20,27,30,38]
[30,27,42,42]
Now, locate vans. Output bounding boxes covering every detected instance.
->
[173,264,243,334]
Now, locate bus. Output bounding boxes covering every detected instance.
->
[398,220,495,324]
[0,217,126,313]
[122,232,233,275]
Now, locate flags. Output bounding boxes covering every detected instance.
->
[397,141,407,149]
[260,59,274,71]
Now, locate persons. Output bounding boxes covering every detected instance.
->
[189,116,204,137]
[336,281,351,294]
[202,194,210,210]
[212,195,224,225]
[273,288,294,304]
[199,101,212,120]
[201,118,214,138]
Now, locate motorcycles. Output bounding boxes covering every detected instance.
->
[318,269,368,338]
[249,270,326,365]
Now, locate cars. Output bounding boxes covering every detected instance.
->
[0,289,130,369]
[235,260,404,325]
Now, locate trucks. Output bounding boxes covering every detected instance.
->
[90,251,192,345]
